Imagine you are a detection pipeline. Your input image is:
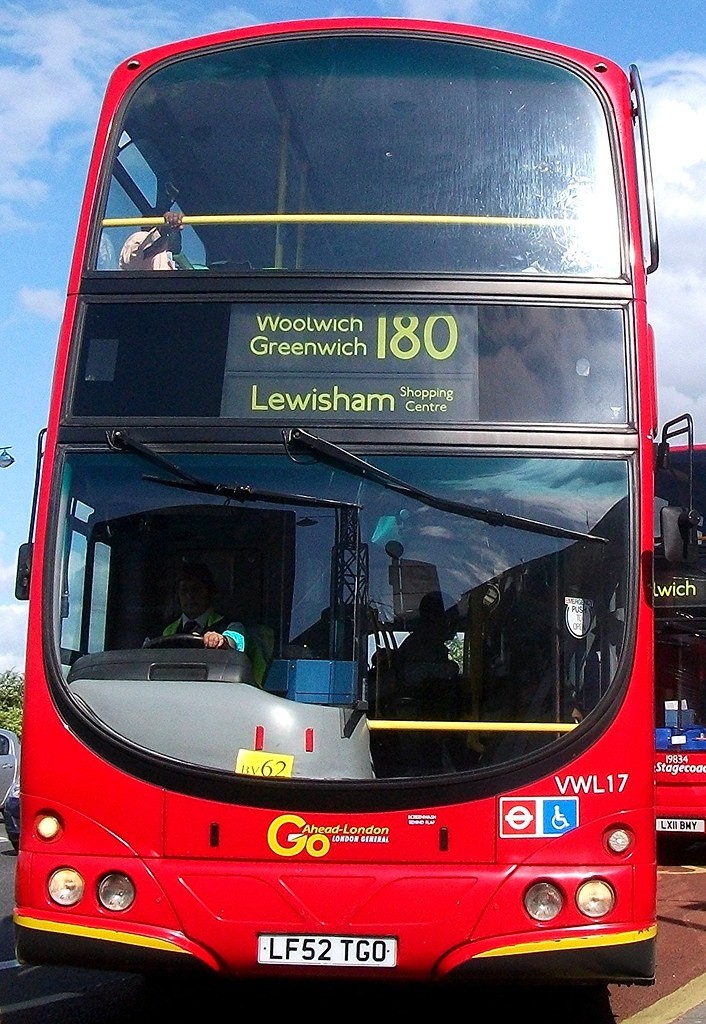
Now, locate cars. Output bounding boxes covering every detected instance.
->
[0,729,22,818]
[4,776,22,853]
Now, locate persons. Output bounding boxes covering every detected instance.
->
[118,206,185,271]
[141,569,249,652]
[390,591,583,776]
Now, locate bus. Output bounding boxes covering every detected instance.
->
[6,13,698,988]
[645,442,706,850]
